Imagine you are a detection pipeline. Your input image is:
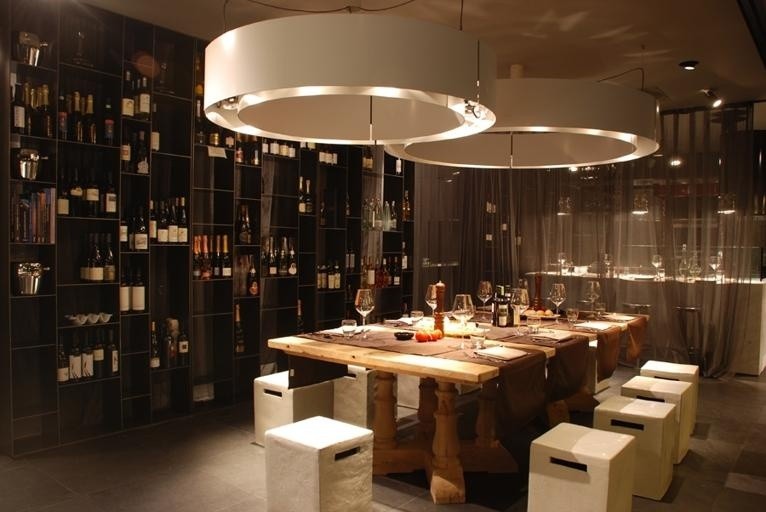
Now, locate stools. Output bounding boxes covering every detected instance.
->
[593,395,677,500]
[616,302,652,368]
[263,414,373,512]
[674,305,703,365]
[622,375,693,466]
[639,359,704,437]
[588,340,610,395]
[526,422,635,512]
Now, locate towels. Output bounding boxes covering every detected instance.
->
[534,325,572,342]
[573,317,613,331]
[472,344,526,360]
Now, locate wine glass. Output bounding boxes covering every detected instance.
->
[355,278,602,349]
[557,252,722,282]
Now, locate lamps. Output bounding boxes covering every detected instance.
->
[379,38,660,170]
[530,56,738,221]
[200,0,497,147]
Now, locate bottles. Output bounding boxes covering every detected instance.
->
[316,256,343,290]
[196,100,207,143]
[298,299,305,334]
[238,202,253,246]
[402,241,408,271]
[236,251,260,297]
[121,125,150,174]
[403,190,410,222]
[58,158,116,219]
[193,231,231,277]
[58,74,118,146]
[57,329,120,383]
[383,254,401,285]
[262,232,298,276]
[385,198,397,231]
[320,199,326,226]
[345,182,351,215]
[123,71,151,121]
[235,304,246,357]
[263,137,298,157]
[151,194,188,244]
[11,72,54,138]
[151,102,162,150]
[679,243,691,276]
[394,157,402,176]
[151,316,190,368]
[298,176,314,213]
[299,140,316,150]
[210,126,235,149]
[195,54,204,97]
[120,202,149,252]
[318,145,339,166]
[120,253,148,314]
[81,227,116,281]
